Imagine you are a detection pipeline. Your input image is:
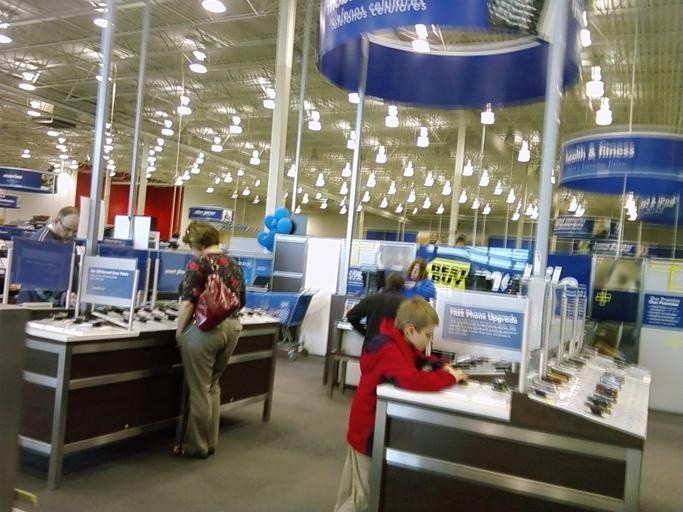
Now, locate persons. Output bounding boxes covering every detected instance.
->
[331,294,467,512]
[17,205,76,308]
[594,257,642,292]
[345,270,404,359]
[401,258,436,302]
[163,220,243,458]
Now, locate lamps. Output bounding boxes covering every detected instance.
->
[0,0,638,226]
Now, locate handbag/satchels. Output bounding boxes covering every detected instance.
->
[194,275,241,331]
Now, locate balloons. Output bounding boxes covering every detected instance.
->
[255,206,292,252]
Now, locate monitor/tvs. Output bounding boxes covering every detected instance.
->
[362,269,385,290]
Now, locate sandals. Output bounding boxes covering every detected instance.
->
[172,444,214,459]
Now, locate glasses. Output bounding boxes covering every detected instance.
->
[57,219,77,235]
